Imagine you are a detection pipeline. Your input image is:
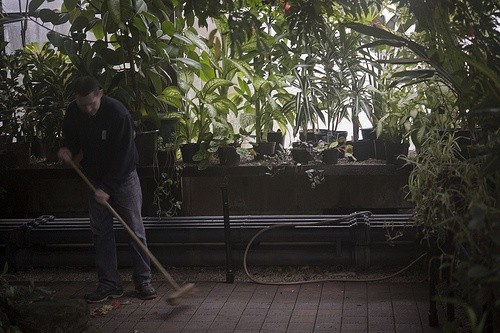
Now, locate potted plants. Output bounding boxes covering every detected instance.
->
[0,15,489,166]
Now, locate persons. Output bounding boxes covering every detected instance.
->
[57,75,157,303]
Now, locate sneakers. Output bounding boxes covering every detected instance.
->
[85,282,123,303]
[133,283,158,300]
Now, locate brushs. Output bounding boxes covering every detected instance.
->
[58,145,202,308]
